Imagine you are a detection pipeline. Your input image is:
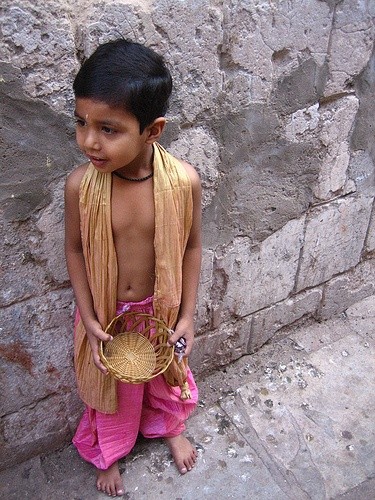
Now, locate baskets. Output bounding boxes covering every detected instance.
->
[98,311,174,384]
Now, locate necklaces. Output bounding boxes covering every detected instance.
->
[112,172,153,183]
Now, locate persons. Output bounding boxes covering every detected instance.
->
[63,40,202,497]
